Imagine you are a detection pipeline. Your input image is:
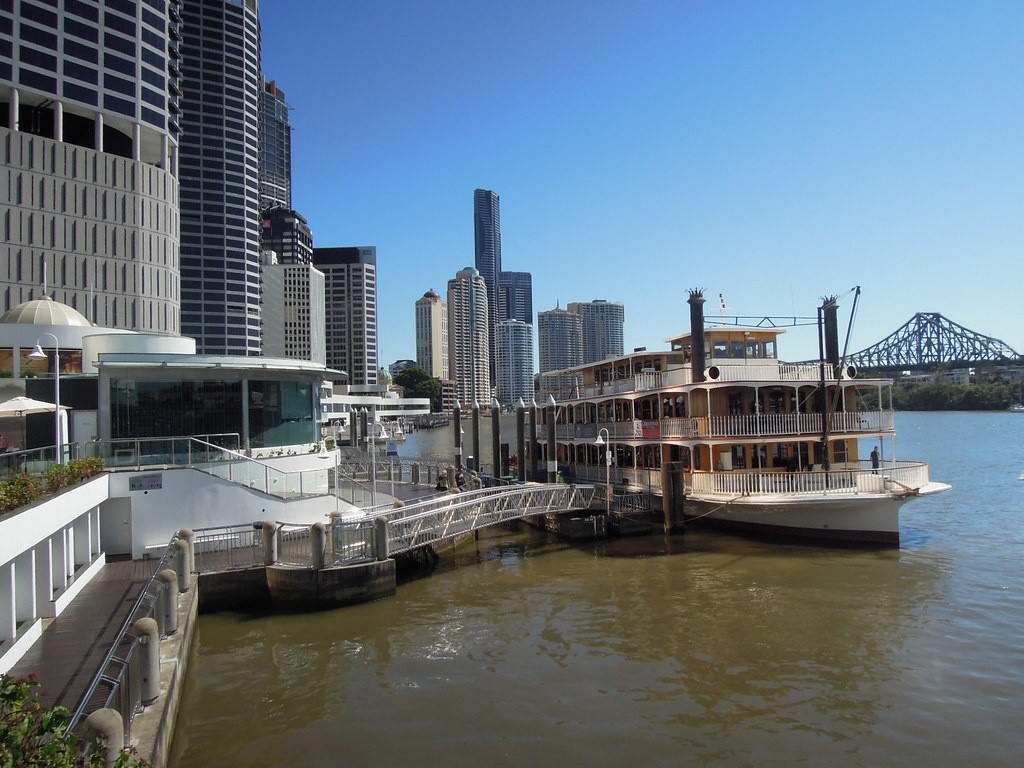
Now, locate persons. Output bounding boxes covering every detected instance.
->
[557,470,565,483]
[435,466,485,497]
[786,453,806,485]
[0,434,24,473]
[510,454,519,465]
[870,445,880,474]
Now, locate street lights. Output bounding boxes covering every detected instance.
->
[318,435,338,512]
[593,429,611,503]
[333,421,344,495]
[371,423,388,507]
[29,333,59,471]
[389,421,404,501]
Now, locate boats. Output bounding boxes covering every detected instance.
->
[415,413,450,428]
[508,286,951,544]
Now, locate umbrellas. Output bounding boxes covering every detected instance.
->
[0,396,74,450]
